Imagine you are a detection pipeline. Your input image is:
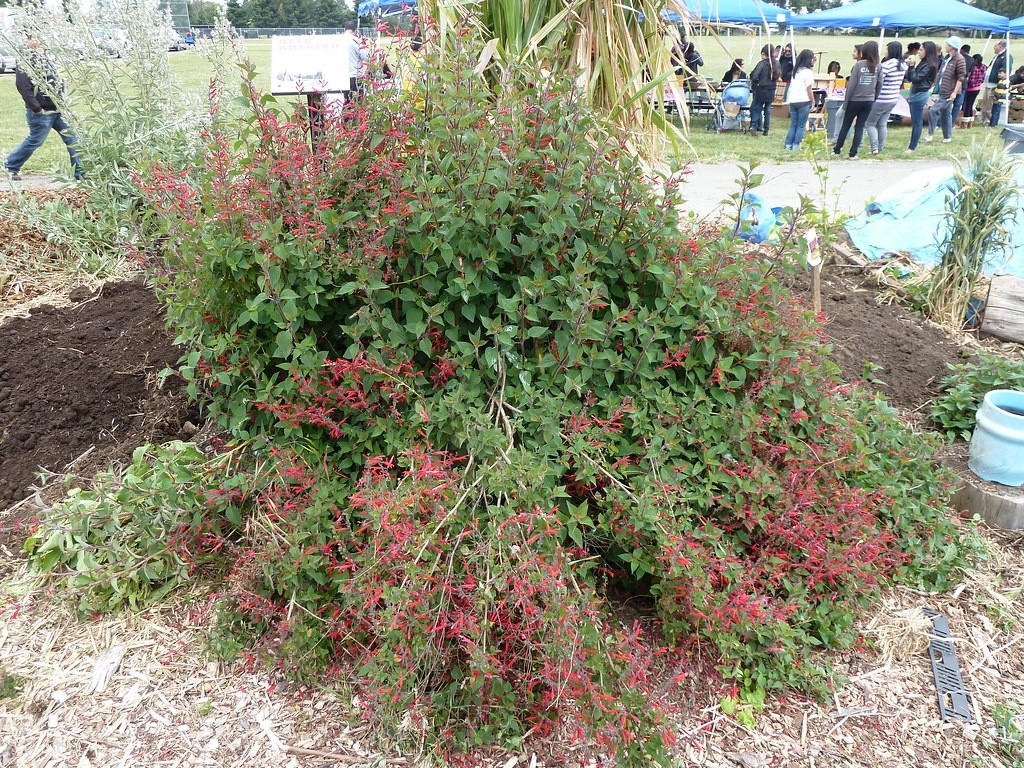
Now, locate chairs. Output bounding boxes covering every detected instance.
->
[806,81,835,131]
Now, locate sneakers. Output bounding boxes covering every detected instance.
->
[10,172,22,181]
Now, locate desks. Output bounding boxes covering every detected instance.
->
[683,84,754,93]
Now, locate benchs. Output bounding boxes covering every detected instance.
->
[648,101,773,109]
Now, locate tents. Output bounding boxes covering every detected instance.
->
[601,1,1024,124]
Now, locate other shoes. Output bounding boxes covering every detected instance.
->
[942,137,952,142]
[924,135,932,141]
[830,152,839,156]
[750,128,757,136]
[849,155,860,160]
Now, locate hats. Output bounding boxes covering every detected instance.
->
[946,35,962,49]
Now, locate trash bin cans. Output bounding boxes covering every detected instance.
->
[826,96,854,139]
[1001,125,1024,155]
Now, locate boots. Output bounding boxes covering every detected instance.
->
[959,117,974,129]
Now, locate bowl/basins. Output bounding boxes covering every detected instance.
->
[686,82,702,89]
[903,83,912,90]
[836,78,845,89]
[708,82,720,88]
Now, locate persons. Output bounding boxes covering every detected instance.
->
[341,20,371,130]
[665,33,1024,161]
[0,25,93,181]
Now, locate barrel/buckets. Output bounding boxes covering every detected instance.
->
[775,82,787,100]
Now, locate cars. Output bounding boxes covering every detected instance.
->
[0,31,187,73]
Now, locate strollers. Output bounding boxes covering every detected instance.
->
[804,86,828,133]
[707,80,761,137]
